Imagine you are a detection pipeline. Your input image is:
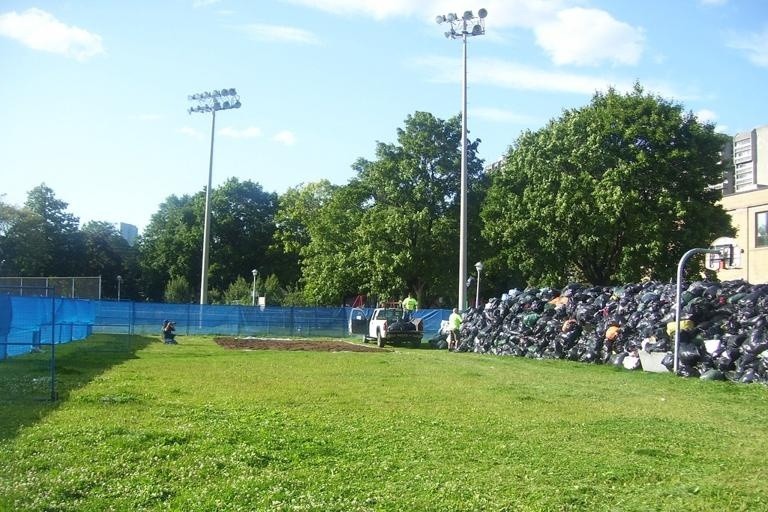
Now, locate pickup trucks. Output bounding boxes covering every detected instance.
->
[348,307,424,347]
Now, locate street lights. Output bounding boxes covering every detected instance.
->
[474,262,483,308]
[435,8,488,311]
[251,269,258,306]
[187,88,242,329]
[117,276,121,302]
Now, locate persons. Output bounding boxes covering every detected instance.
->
[448,307,466,351]
[401,292,419,321]
[161,318,177,343]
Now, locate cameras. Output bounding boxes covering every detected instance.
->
[170,321,176,326]
